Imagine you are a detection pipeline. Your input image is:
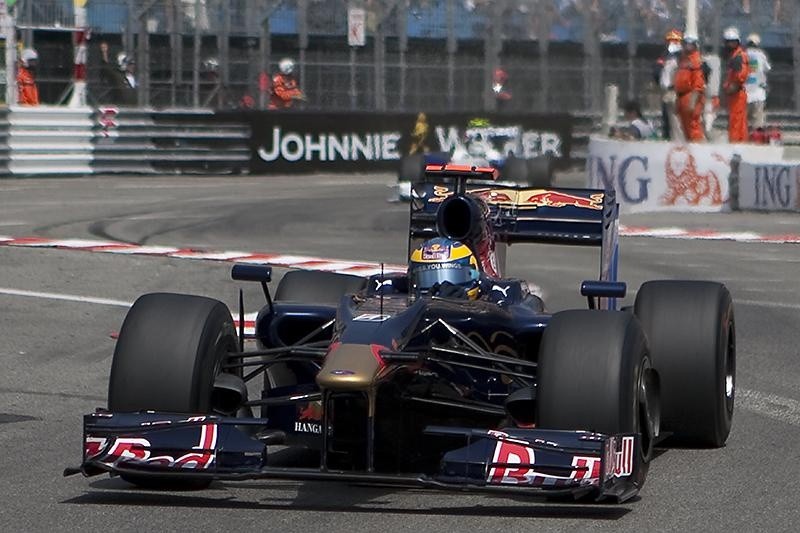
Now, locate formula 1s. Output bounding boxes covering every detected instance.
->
[61,162,738,502]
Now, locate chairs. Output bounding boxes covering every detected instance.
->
[367,272,523,306]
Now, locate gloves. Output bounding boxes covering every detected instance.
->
[725,82,740,96]
[432,281,469,301]
[688,92,698,111]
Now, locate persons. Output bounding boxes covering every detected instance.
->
[14,47,42,107]
[353,236,520,398]
[266,56,307,111]
[653,28,773,144]
[610,102,651,144]
[102,41,142,106]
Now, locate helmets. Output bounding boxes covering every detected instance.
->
[747,34,760,47]
[199,59,219,75]
[116,53,136,72]
[278,58,294,76]
[407,237,481,303]
[666,30,682,41]
[682,35,700,49]
[20,48,38,68]
[723,29,742,44]
